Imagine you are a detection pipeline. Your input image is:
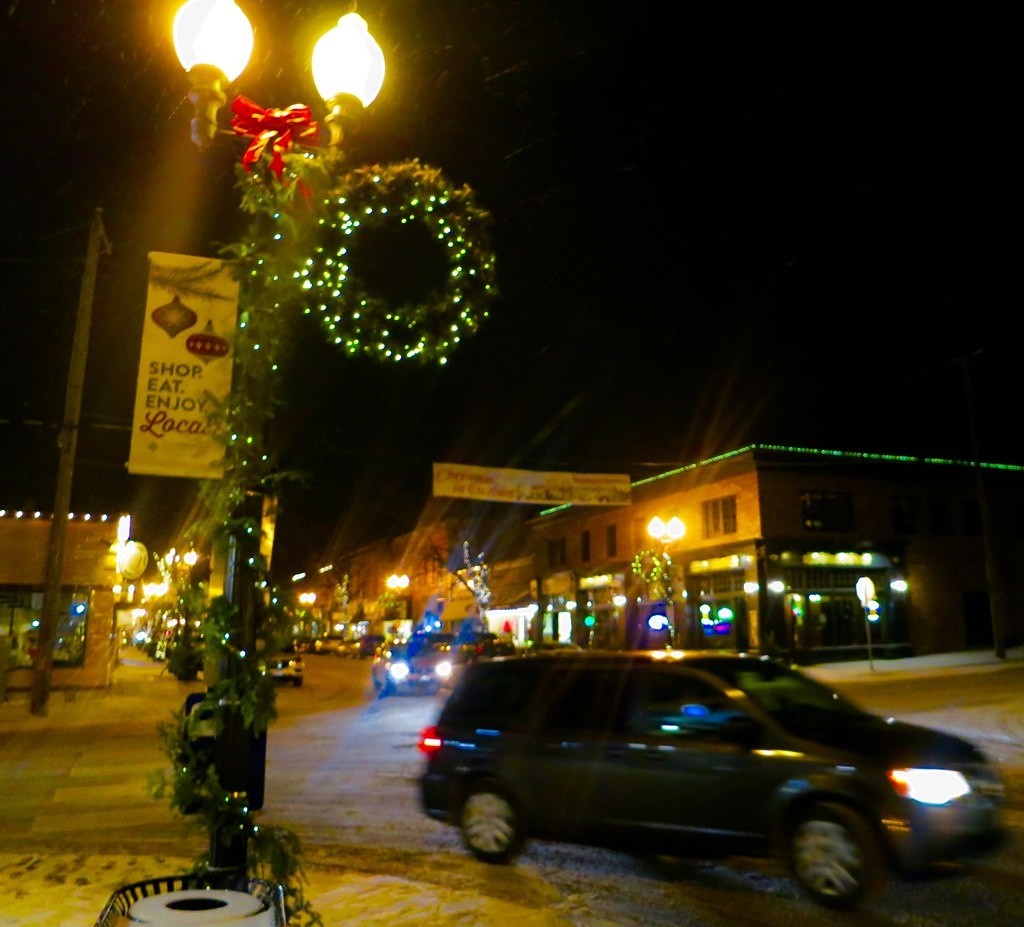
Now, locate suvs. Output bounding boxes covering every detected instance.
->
[413,646,1007,911]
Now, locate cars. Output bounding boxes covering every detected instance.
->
[268,642,303,686]
[374,643,448,698]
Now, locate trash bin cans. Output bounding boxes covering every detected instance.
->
[93,874,287,927]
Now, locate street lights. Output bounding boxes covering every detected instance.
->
[170,0,386,884]
[179,541,197,678]
[649,515,686,646]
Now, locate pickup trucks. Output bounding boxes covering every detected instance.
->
[409,631,517,666]
